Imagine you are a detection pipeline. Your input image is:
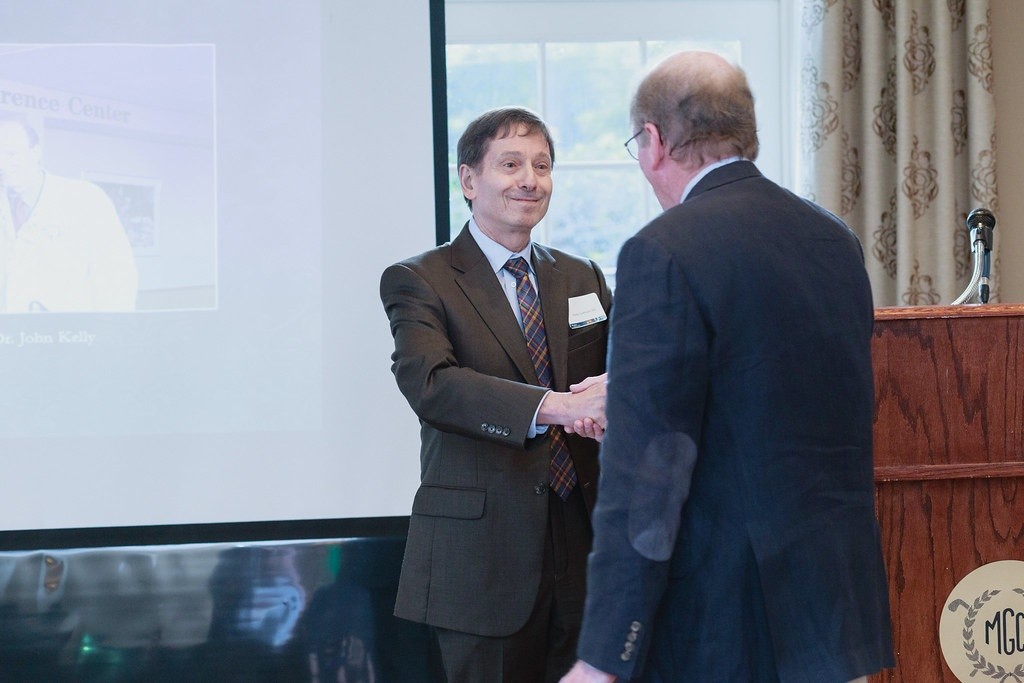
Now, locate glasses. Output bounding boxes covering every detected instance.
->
[624,121,663,160]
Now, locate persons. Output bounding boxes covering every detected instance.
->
[380,107,617,683]
[1,84,141,314]
[555,50,897,683]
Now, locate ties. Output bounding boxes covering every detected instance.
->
[503,256,578,504]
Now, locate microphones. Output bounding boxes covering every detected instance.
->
[967,208,996,302]
